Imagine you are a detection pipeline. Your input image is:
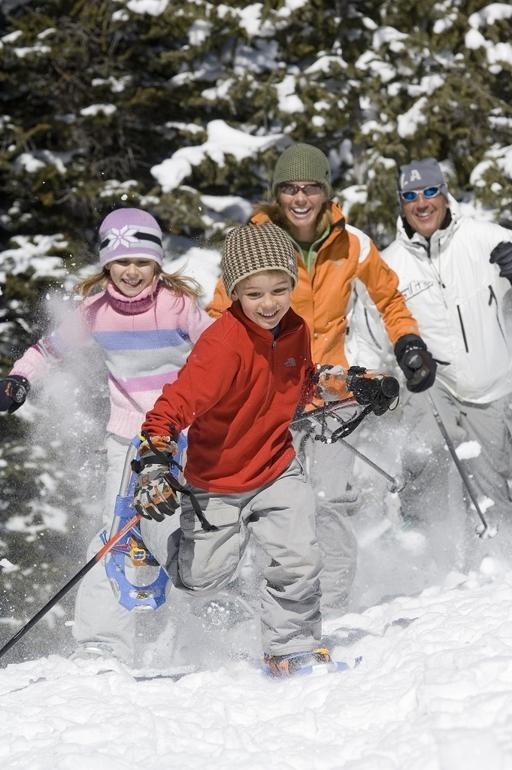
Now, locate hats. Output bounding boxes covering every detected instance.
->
[273,143,333,205]
[99,208,163,271]
[398,157,447,200]
[222,223,298,302]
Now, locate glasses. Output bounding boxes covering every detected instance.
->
[279,182,325,197]
[402,184,444,201]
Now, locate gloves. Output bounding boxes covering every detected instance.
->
[394,334,436,392]
[490,242,512,281]
[131,436,181,521]
[0,375,31,415]
[346,375,396,416]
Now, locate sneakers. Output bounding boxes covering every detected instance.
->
[264,648,329,676]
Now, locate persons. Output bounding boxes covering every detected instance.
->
[346,158,512,590]
[131,221,347,680]
[203,141,437,647]
[0,207,217,674]
